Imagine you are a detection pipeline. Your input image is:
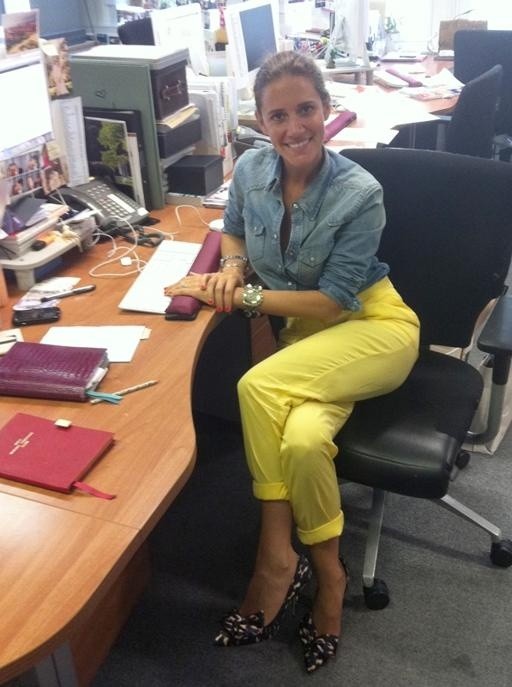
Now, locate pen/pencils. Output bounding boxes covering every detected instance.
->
[89,380,158,404]
[41,284,96,302]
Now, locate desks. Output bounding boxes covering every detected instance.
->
[316,47,466,153]
[0,202,261,687]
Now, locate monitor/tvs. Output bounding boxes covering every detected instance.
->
[327,0,366,68]
[0,49,53,161]
[225,0,283,90]
[149,2,209,81]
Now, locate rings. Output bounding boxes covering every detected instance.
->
[181,284,188,288]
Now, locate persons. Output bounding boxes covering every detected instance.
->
[9,158,36,195]
[163,46,423,674]
[48,56,70,96]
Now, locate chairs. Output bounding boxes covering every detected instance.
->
[432,62,504,160]
[336,147,511,612]
[447,28,512,162]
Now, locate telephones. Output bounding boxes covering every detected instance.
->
[233,125,271,155]
[47,179,150,239]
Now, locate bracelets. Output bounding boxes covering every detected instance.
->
[219,255,248,272]
[241,284,263,320]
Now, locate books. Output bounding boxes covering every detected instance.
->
[85,106,153,213]
[0,341,110,402]
[0,413,116,494]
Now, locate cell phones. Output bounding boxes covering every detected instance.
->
[12,305,61,325]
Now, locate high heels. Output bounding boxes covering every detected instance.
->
[212,552,313,646]
[298,554,350,674]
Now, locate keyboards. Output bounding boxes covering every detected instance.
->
[116,238,203,315]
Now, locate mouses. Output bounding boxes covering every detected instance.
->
[208,217,224,232]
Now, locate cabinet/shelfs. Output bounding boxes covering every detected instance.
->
[68,43,225,211]
